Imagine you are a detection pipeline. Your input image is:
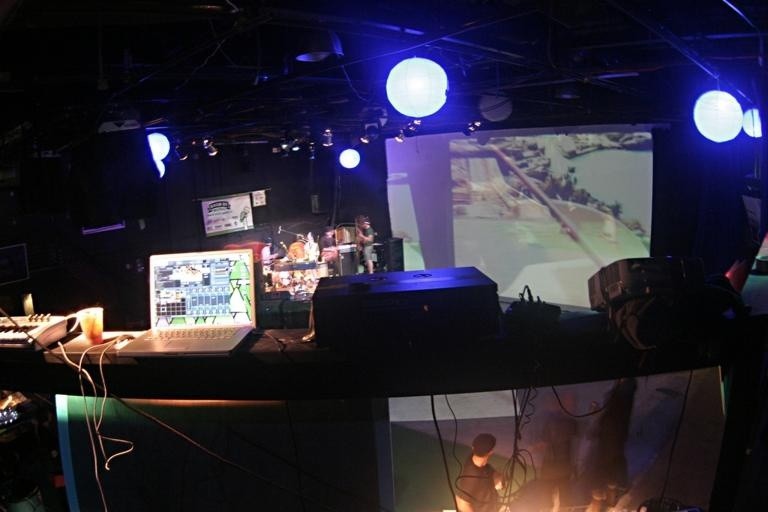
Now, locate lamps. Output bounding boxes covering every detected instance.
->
[319,117,489,147]
[171,132,218,160]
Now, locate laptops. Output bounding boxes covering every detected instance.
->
[117,247,257,358]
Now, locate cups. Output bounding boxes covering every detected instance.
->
[74,306,105,346]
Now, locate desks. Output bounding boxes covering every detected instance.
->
[42,321,746,510]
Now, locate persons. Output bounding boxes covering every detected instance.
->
[261,216,374,276]
[455,434,505,512]
[537,377,638,490]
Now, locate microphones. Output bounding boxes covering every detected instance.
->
[277,226,282,234]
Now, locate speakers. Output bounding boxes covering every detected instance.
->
[373,243,384,271]
[383,237,404,272]
[335,243,358,277]
